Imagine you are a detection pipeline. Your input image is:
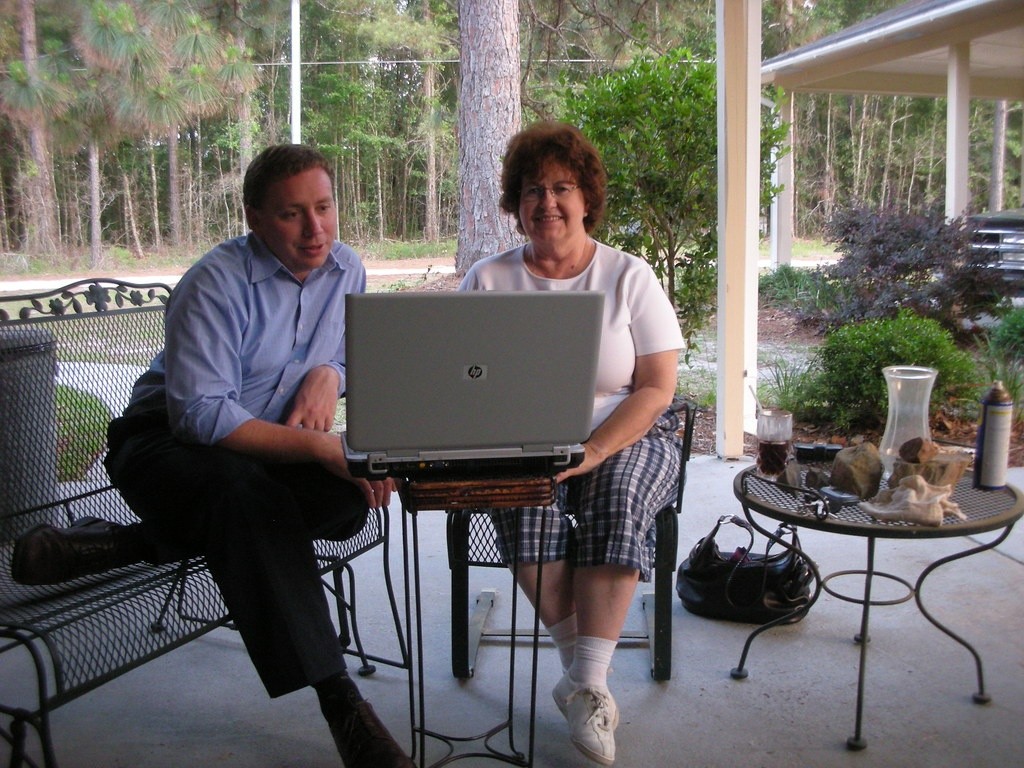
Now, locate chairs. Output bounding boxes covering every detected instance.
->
[447,398,699,680]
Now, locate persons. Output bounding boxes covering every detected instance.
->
[455,122,689,766]
[11,145,415,768]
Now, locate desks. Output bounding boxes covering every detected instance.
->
[394,475,557,767]
[731,457,1024,749]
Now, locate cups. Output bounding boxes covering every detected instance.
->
[758,409,792,481]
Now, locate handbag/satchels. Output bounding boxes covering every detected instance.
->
[675,514,818,625]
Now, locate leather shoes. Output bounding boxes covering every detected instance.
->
[328,698,416,768]
[10,517,137,585]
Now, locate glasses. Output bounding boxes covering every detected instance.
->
[517,180,582,202]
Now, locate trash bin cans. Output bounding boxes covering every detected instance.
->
[0,326,59,543]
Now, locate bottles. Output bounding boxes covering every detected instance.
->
[877,365,939,482]
[972,380,1012,491]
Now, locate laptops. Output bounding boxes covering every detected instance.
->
[342,292,606,475]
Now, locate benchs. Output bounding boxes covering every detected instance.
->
[1,277,409,768]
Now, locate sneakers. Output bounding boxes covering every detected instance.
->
[563,668,619,731]
[552,670,616,766]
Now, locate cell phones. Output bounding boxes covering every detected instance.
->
[819,486,860,503]
